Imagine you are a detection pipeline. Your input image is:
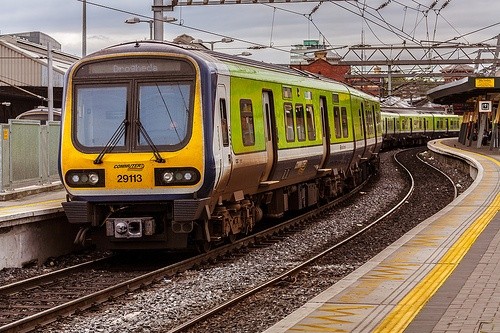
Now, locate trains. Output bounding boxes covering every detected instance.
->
[58,39,464,244]
